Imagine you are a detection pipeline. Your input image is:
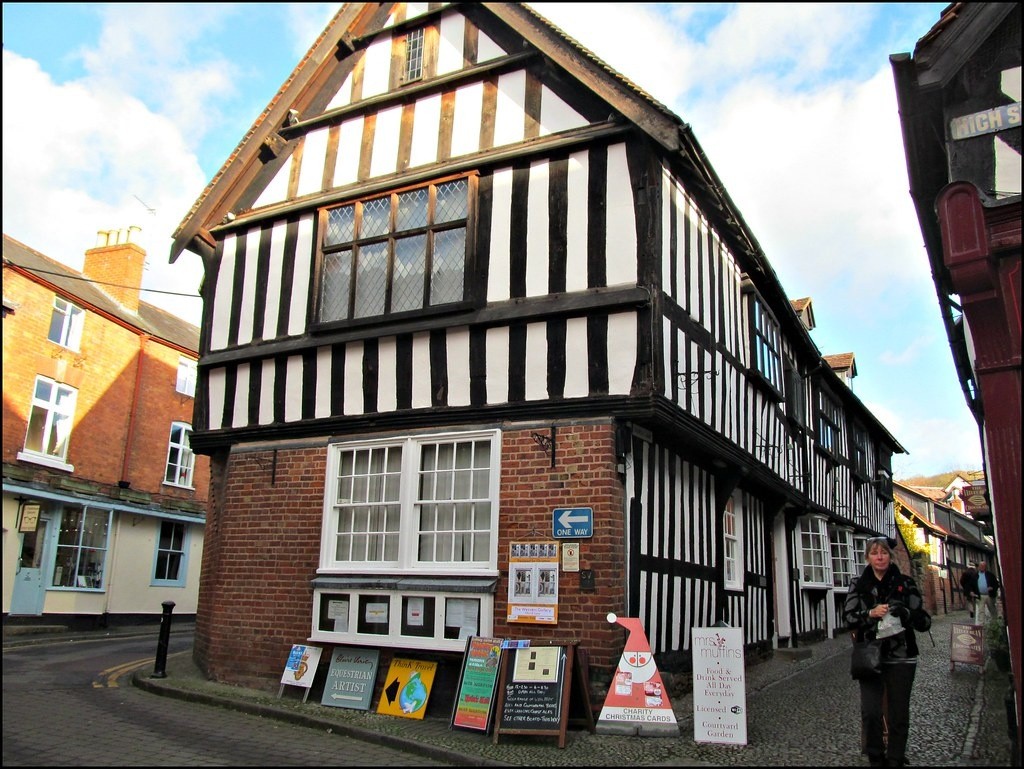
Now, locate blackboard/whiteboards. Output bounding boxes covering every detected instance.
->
[494,641,593,735]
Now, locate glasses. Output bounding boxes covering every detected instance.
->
[867,536,887,545]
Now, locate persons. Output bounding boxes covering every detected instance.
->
[960,562,978,617]
[845,538,932,766]
[967,561,998,626]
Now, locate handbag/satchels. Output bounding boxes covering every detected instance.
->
[851,641,882,675]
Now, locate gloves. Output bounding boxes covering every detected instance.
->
[890,602,908,620]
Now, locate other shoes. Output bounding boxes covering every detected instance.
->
[869,752,885,767]
[887,757,903,768]
